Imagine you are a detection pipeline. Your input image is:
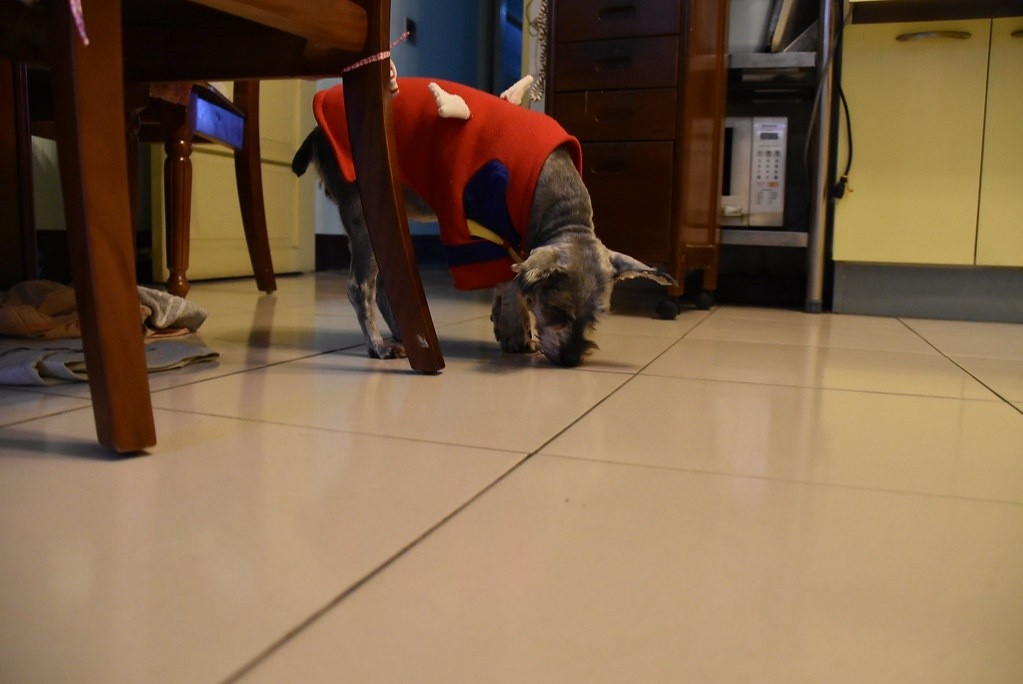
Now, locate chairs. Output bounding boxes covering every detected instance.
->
[0,0,450,454]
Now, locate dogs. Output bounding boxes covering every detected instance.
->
[289,78,679,368]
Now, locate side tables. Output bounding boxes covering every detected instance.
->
[149,75,319,284]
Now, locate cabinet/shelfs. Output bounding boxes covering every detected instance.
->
[725,0,842,316]
[833,1,1023,326]
[542,0,731,315]
